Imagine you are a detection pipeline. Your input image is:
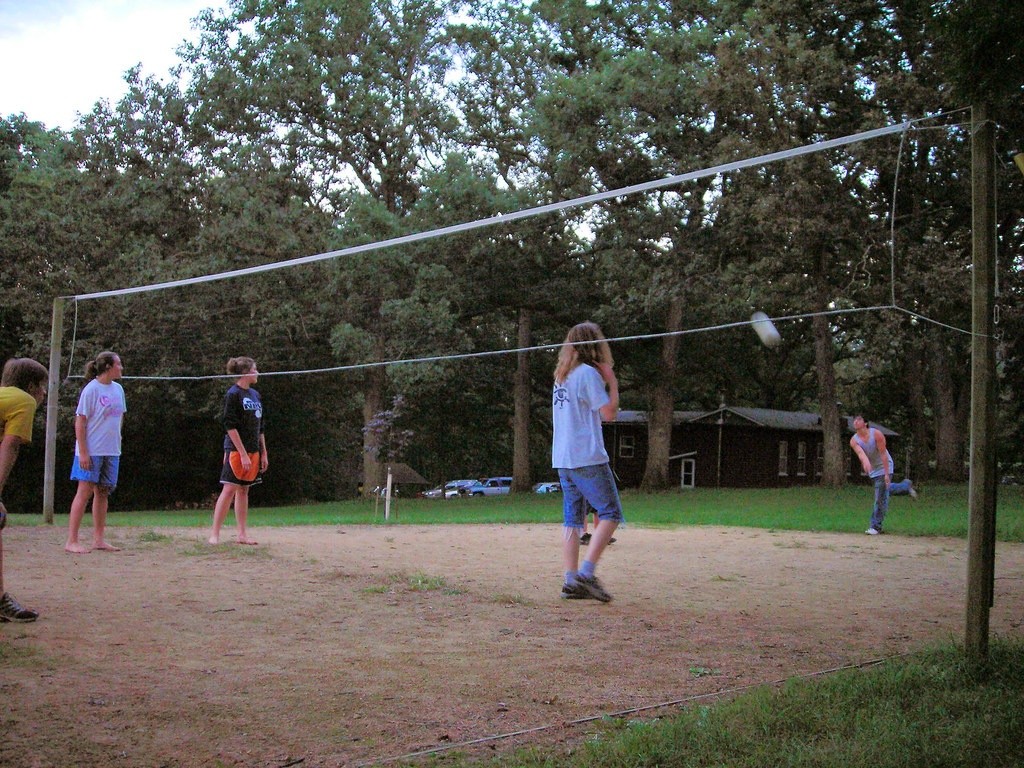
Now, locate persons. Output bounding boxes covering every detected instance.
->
[0,359,49,623]
[849,414,916,536]
[209,356,268,546]
[63,352,128,554]
[552,323,623,603]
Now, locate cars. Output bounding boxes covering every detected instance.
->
[423,476,561,497]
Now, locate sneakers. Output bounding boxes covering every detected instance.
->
[573,574,611,602]
[560,582,593,599]
[0,592,39,623]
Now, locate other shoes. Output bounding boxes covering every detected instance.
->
[905,479,917,498]
[608,537,617,545]
[579,533,592,545]
[865,527,878,535]
